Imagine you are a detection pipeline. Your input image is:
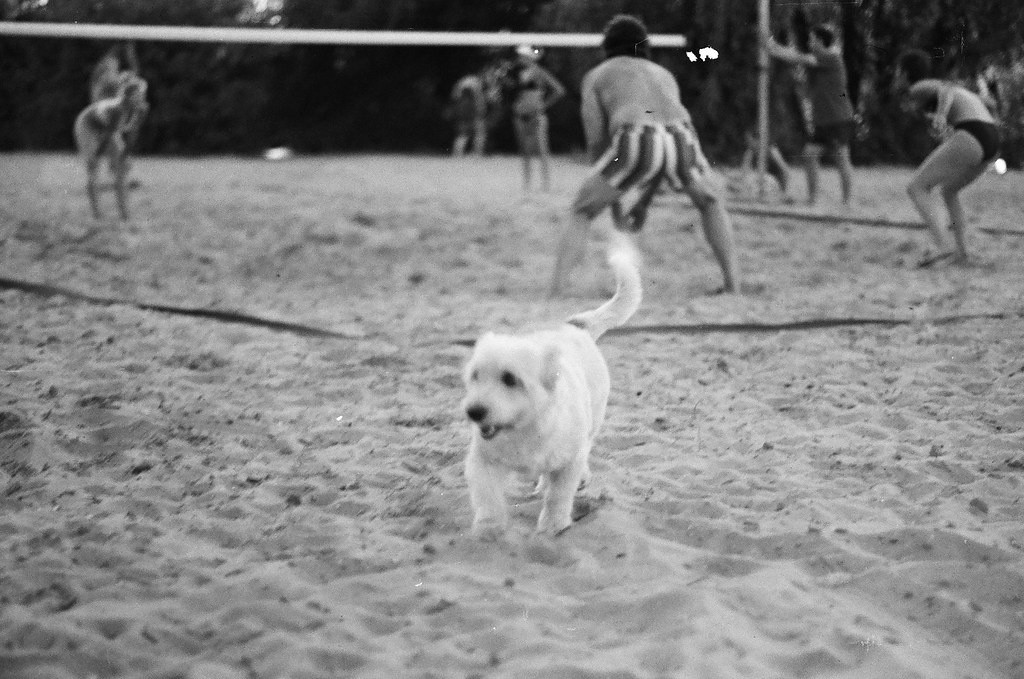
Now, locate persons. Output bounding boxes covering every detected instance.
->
[747,25,860,207]
[74,34,149,220]
[545,13,743,305]
[452,43,566,198]
[894,49,1001,268]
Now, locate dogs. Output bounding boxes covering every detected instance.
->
[456,243,645,543]
